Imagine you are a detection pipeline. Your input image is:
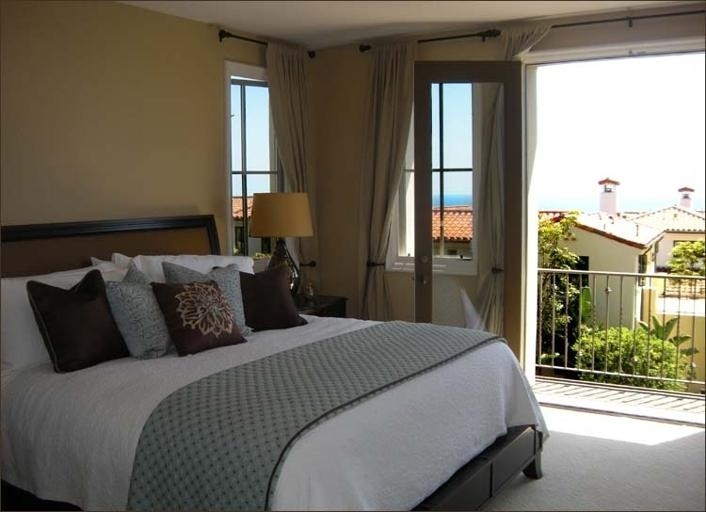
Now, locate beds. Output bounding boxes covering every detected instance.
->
[0,214,544,511]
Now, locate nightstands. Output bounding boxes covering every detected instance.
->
[293,293,349,318]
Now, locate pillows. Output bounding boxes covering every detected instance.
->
[148,276,250,356]
[104,259,169,357]
[159,259,254,338]
[209,262,308,332]
[24,268,127,373]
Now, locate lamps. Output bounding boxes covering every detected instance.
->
[247,192,314,299]
